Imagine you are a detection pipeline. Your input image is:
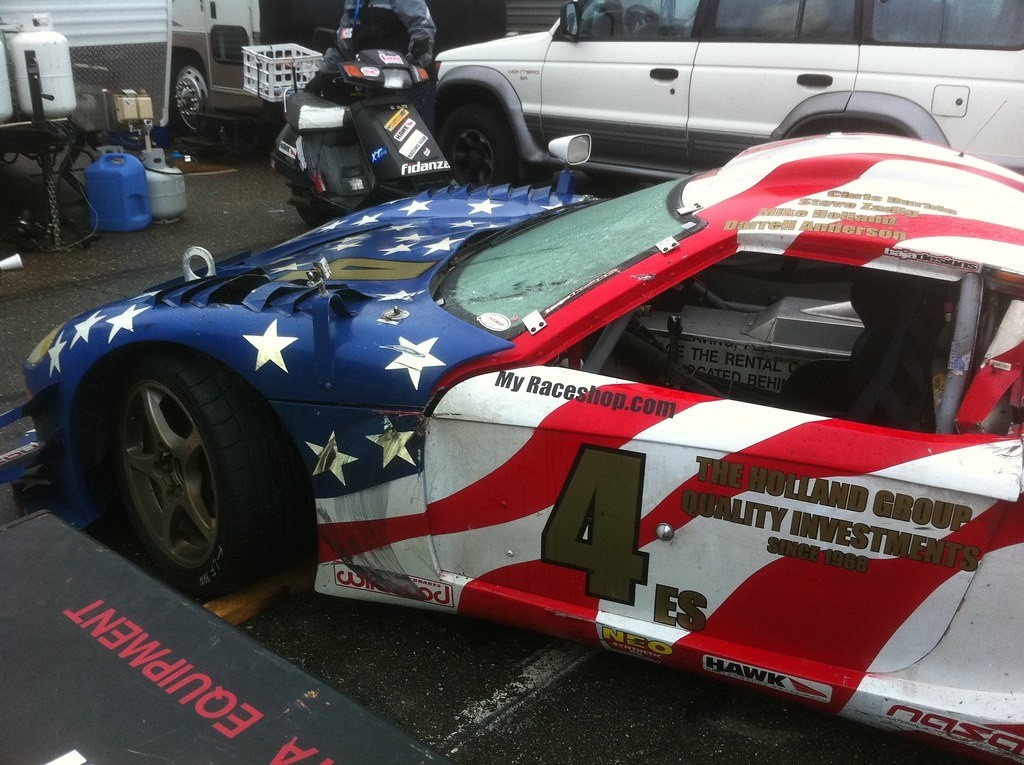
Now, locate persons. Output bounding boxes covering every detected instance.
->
[304,0,437,96]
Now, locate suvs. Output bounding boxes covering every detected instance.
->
[432,0,1024,192]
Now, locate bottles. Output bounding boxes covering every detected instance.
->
[83,153,151,232]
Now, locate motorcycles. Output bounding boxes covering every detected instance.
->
[270,45,457,227]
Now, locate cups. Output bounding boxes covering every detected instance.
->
[0,253,24,270]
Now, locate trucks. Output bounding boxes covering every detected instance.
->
[163,0,344,129]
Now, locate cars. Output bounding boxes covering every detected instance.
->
[19,133,1024,765]
